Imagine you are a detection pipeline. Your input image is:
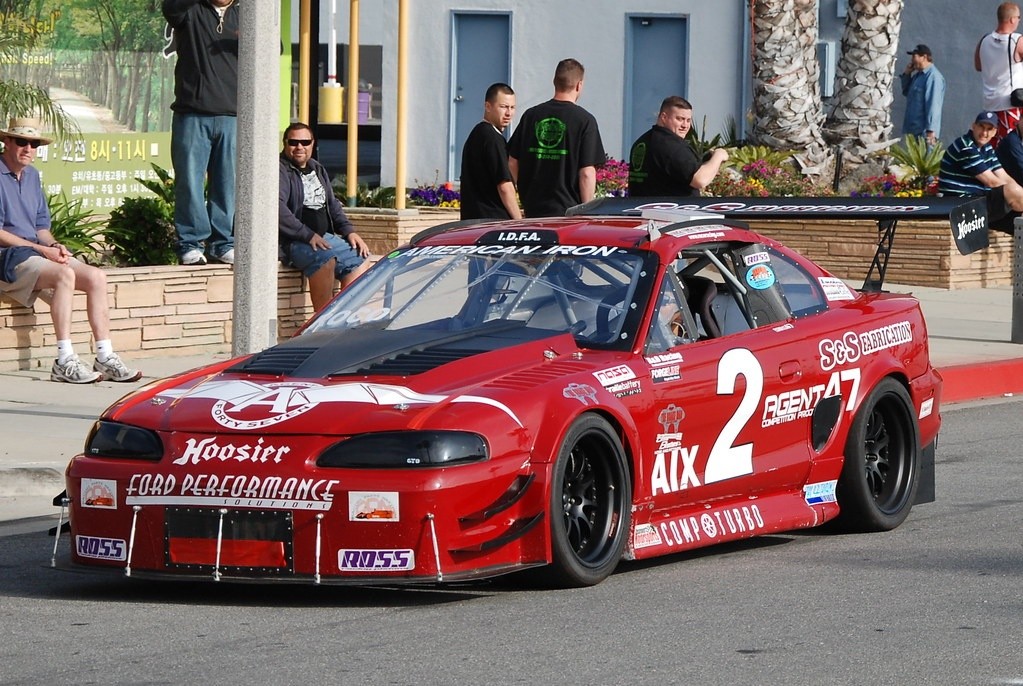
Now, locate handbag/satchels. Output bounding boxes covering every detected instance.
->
[1011,88,1023,108]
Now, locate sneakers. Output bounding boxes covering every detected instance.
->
[177,249,209,265]
[209,245,235,264]
[51,353,104,384]
[92,353,143,382]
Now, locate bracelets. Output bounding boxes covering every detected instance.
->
[49,242,57,247]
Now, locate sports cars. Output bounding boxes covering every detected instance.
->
[49,194,990,589]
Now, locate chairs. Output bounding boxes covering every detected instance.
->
[596,274,720,348]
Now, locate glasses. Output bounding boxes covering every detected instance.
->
[9,136,40,149]
[284,137,313,147]
[661,295,678,307]
[1009,15,1021,20]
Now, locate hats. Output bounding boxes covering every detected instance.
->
[0,118,55,146]
[906,44,932,55]
[975,111,999,128]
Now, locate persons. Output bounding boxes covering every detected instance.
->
[996,113,1023,188]
[279,122,371,312]
[628,95,729,275]
[899,45,945,182]
[506,58,606,277]
[974,2,1023,150]
[162,0,283,265]
[460,83,521,295]
[937,110,1023,235]
[0,118,142,385]
